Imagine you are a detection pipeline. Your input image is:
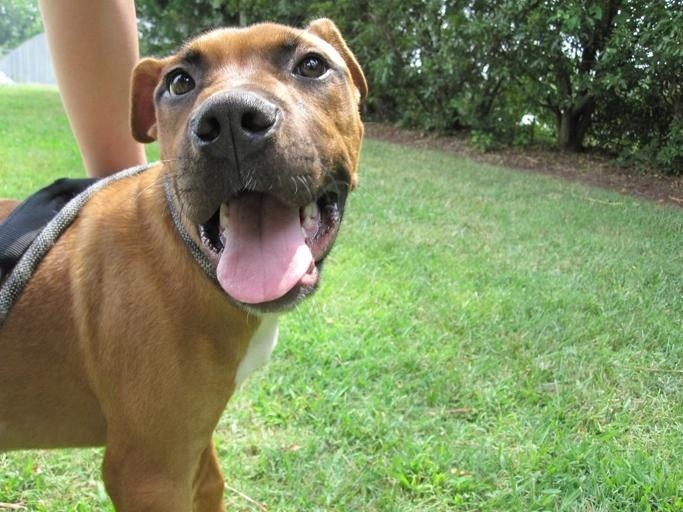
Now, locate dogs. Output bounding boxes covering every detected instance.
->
[0,15,369,512]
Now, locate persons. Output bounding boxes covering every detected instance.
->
[36,0,147,177]
